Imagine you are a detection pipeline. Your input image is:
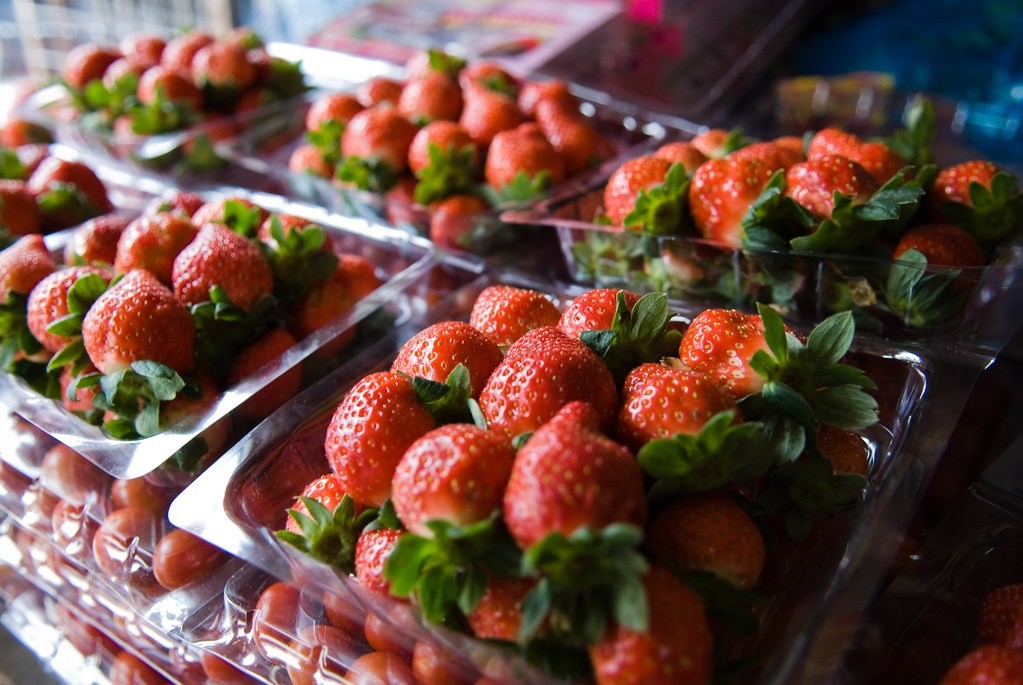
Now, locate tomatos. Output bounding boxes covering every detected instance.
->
[0,401,531,685]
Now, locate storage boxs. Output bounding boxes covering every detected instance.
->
[1,41,1022,685]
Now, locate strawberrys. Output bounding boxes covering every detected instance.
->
[269,124,1023,685]
[0,27,646,489]
